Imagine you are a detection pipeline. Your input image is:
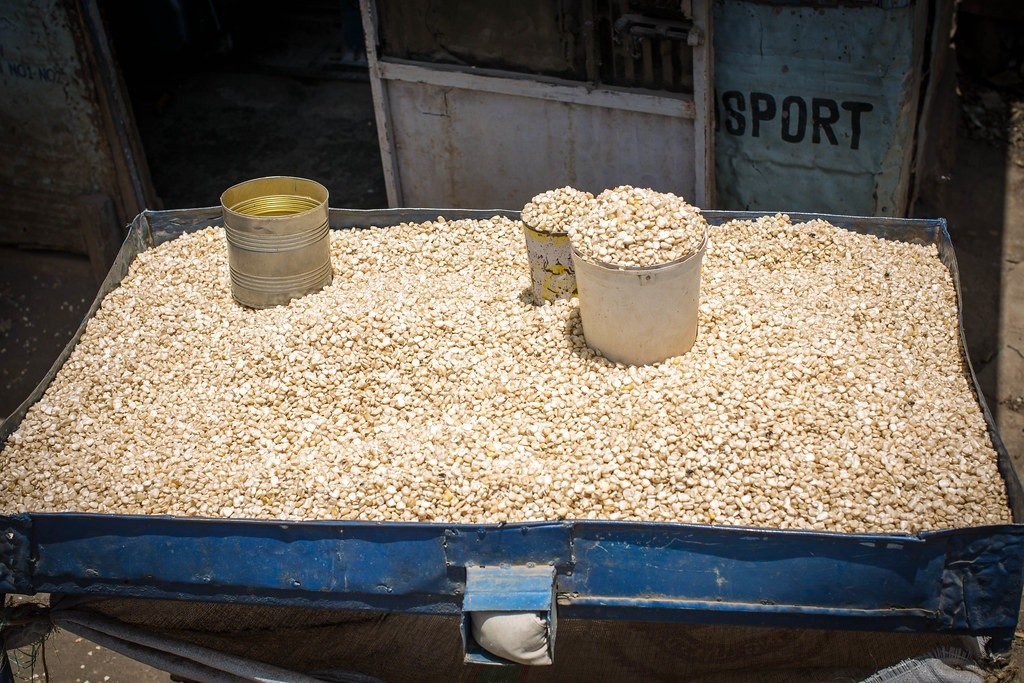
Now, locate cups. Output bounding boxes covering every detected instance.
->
[221,175,333,309]
[570,227,708,367]
[520,216,578,306]
